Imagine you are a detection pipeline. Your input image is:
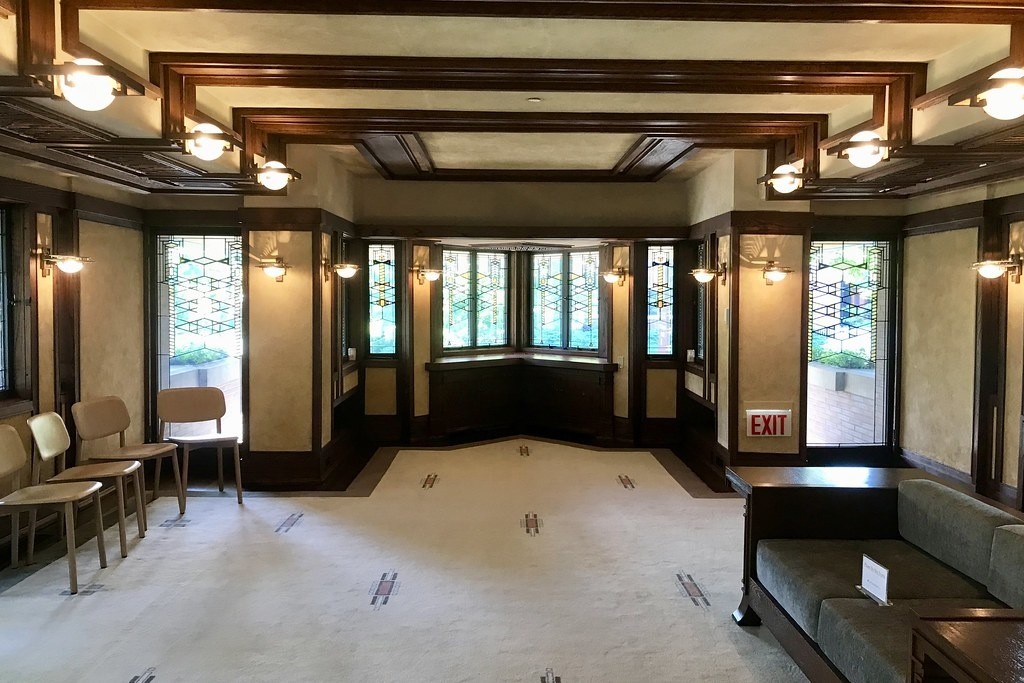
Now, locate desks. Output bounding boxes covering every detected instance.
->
[907,604,1024,683]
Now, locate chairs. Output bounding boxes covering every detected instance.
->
[0,425,107,595]
[72,395,185,532]
[28,411,146,558]
[152,387,243,509]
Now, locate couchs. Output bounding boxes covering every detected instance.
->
[720,464,1024,682]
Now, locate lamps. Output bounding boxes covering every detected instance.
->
[17,57,145,111]
[40,247,96,278]
[165,123,233,162]
[761,261,794,286]
[688,262,726,286]
[826,130,907,169]
[324,258,361,283]
[255,257,292,282]
[948,66,1024,120]
[755,164,817,193]
[243,160,302,191]
[599,267,626,286]
[969,236,1022,285]
[414,265,444,285]
